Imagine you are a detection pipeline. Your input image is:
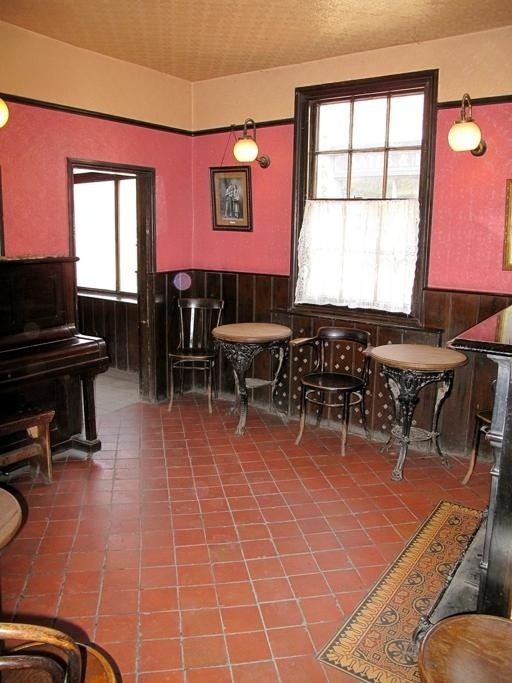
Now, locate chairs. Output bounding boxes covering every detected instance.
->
[290,325,371,458]
[462,380,497,485]
[0,623,121,683]
[166,298,226,415]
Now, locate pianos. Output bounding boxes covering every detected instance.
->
[1,255,109,478]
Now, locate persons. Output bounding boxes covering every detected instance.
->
[231,184,241,219]
[224,183,234,217]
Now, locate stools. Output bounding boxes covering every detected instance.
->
[0,403,56,482]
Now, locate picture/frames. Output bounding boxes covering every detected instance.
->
[501,179,512,272]
[208,164,253,232]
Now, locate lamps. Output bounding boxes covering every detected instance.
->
[234,118,269,168]
[448,92,487,157]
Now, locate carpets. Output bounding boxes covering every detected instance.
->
[316,498,483,683]
